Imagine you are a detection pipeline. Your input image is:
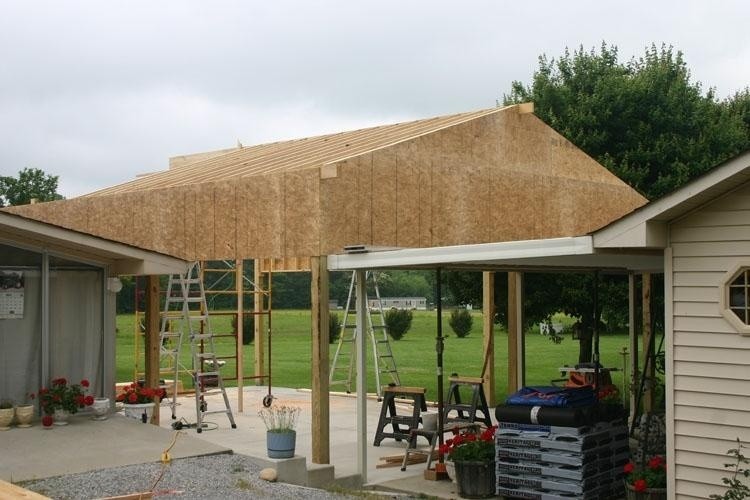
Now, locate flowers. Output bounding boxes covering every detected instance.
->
[35,377,94,412]
[622,447,667,492]
[117,381,165,404]
[437,421,496,461]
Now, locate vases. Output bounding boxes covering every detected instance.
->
[451,456,497,497]
[52,406,68,427]
[618,477,664,500]
[90,397,110,419]
[123,403,156,424]
[420,410,437,431]
[15,404,34,428]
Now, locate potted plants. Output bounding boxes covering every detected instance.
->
[0,399,14,432]
[257,402,302,459]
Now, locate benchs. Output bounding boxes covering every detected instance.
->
[400,418,482,470]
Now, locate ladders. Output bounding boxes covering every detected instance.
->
[159,261,236,433]
[328,270,405,402]
